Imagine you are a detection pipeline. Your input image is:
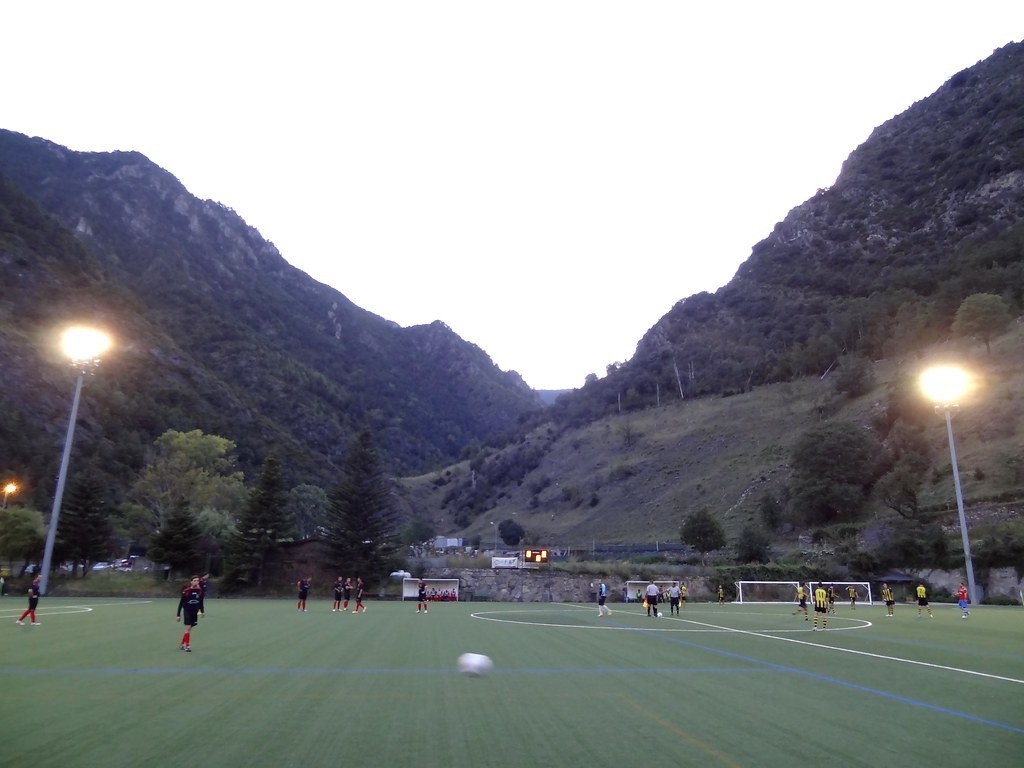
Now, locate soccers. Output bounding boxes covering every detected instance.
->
[657,612,662,617]
[458,652,492,677]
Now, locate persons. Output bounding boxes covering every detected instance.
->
[426,588,456,602]
[792,582,811,621]
[827,584,838,614]
[176,573,210,653]
[15,577,41,625]
[680,582,687,607]
[813,582,828,631]
[719,586,724,605]
[882,584,895,616]
[333,577,353,612]
[298,577,312,611]
[955,583,969,618]
[645,580,681,617]
[915,582,934,618]
[622,584,627,601]
[846,586,859,610]
[598,579,612,617]
[416,577,428,613]
[352,578,366,613]
[637,589,643,603]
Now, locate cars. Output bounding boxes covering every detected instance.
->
[93,561,108,571]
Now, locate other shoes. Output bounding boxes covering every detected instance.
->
[333,609,335,611]
[31,622,41,626]
[930,614,933,617]
[197,611,202,616]
[363,606,366,612]
[352,611,358,613]
[598,613,603,617]
[962,612,970,618]
[344,608,346,610]
[302,609,307,612]
[185,647,191,653]
[424,610,428,613]
[608,611,612,615]
[338,609,342,611]
[886,614,894,616]
[179,645,185,650]
[416,610,421,613]
[15,620,24,625]
[813,627,817,631]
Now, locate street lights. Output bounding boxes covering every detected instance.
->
[36,324,111,596]
[2,483,15,510]
[920,364,978,605]
[490,521,497,557]
